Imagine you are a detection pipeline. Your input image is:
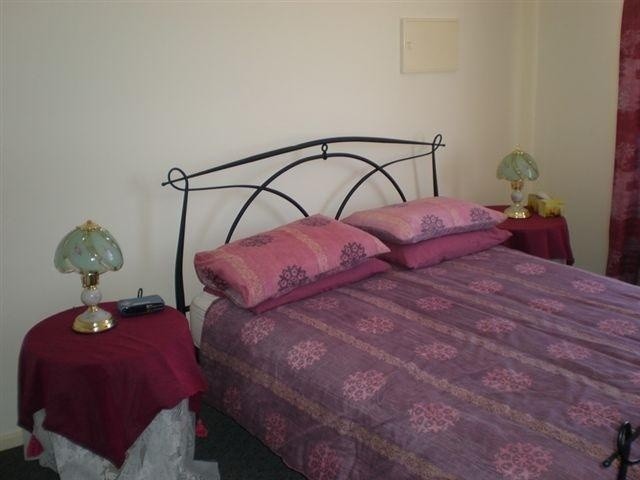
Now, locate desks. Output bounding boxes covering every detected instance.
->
[482,206,570,266]
[23,302,195,480]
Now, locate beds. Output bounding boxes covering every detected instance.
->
[164,135,638,478]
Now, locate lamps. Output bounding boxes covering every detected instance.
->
[53,219,126,335]
[496,143,546,220]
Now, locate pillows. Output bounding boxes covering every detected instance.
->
[378,227,513,270]
[340,197,513,245]
[193,213,392,309]
[203,257,392,315]
